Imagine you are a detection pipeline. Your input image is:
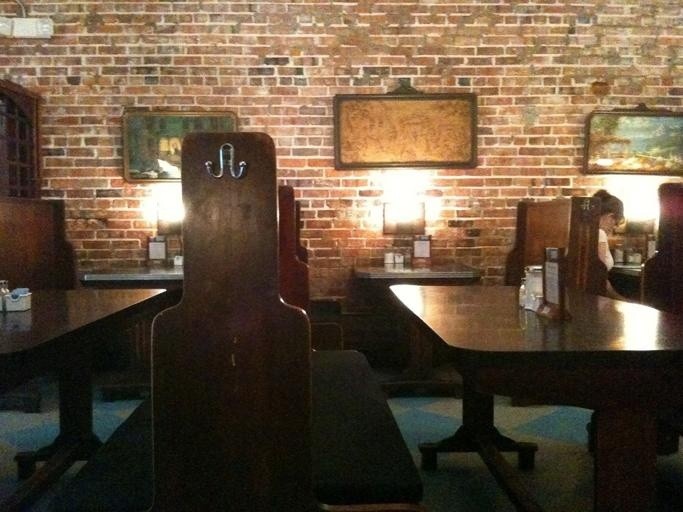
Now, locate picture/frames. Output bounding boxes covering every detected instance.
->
[118,107,239,187]
[581,105,683,179]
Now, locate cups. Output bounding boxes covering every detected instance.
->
[634,254,641,263]
[383,252,403,264]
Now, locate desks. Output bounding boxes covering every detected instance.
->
[0,251,682,512]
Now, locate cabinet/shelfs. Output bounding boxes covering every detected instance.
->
[0,76,71,293]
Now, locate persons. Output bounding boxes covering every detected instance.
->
[593,190,630,301]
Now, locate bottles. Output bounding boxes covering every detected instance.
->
[625,248,634,262]
[404,248,412,265]
[0,280,10,311]
[614,243,625,264]
[524,264,546,310]
[518,278,526,307]
